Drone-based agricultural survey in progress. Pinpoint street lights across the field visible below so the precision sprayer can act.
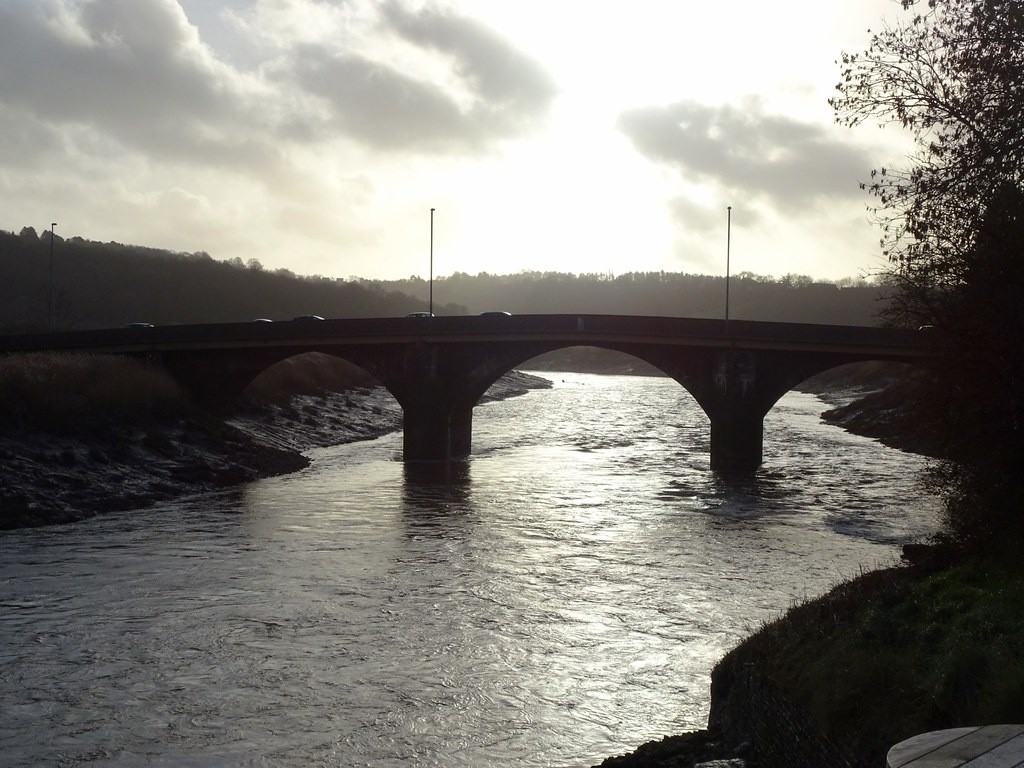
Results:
[48,222,58,332]
[429,208,436,317]
[725,205,733,320]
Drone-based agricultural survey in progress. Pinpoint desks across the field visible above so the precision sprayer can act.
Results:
[884,723,1024,768]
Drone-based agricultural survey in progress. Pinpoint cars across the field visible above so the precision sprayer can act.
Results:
[406,312,435,317]
[253,318,273,322]
[480,311,512,316]
[293,315,326,321]
[121,323,154,327]
